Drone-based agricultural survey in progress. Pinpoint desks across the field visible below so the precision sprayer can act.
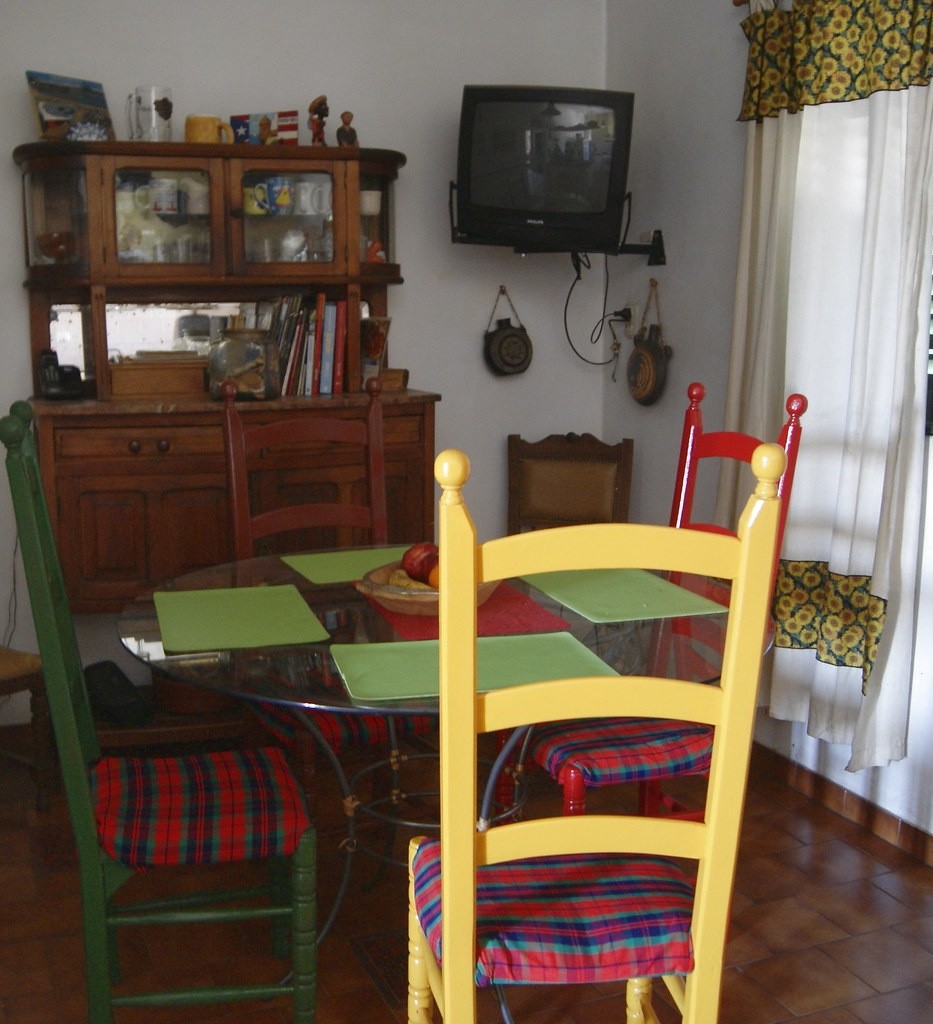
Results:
[113,544,779,1024]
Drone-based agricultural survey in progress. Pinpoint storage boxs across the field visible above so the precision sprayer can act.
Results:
[109,356,208,396]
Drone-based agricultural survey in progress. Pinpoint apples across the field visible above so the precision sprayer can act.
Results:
[401,541,438,585]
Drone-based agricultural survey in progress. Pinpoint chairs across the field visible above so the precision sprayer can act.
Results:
[495,381,809,826]
[219,377,438,821]
[405,441,789,1024]
[508,432,635,537]
[0,400,318,1024]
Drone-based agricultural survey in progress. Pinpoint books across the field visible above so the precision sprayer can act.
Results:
[261,293,393,401]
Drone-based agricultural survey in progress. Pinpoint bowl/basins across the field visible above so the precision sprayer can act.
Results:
[356,559,503,616]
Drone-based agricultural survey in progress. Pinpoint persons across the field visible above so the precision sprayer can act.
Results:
[336,111,359,147]
[308,94,329,146]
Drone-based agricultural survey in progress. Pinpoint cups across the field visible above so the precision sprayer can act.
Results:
[255,238,273,262]
[185,115,234,145]
[360,190,382,215]
[254,176,294,215]
[243,186,268,214]
[126,84,173,142]
[141,231,155,262]
[170,237,190,262]
[199,231,211,263]
[312,182,333,213]
[135,179,178,214]
[291,182,318,215]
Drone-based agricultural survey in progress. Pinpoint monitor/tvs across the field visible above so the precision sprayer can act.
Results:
[454,84,635,249]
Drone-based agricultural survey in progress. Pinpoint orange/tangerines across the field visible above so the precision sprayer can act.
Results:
[387,570,434,590]
[428,565,439,590]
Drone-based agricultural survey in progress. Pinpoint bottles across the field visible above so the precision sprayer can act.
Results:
[323,211,333,260]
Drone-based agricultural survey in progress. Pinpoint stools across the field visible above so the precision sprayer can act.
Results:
[0,645,52,812]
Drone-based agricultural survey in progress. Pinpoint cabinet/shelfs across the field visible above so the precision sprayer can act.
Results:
[11,139,443,794]
